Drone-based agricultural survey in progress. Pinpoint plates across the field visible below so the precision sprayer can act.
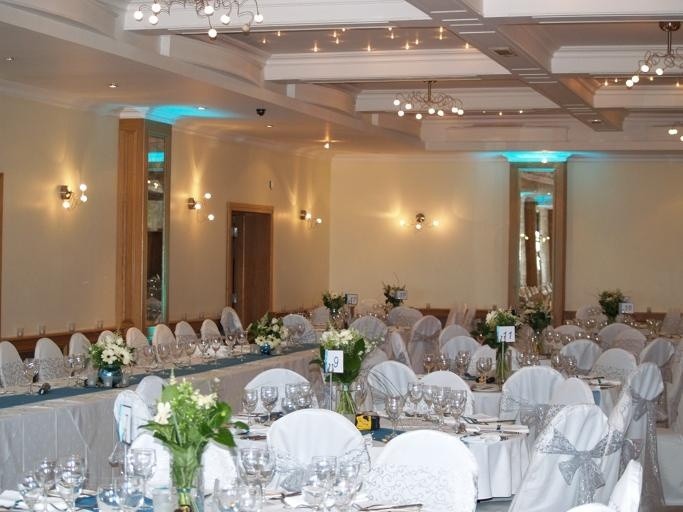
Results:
[457,432,500,444]
[591,384,613,389]
[471,384,499,392]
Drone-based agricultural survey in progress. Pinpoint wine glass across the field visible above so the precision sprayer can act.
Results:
[517,332,542,368]
[127,331,249,380]
[422,350,493,384]
[15,440,176,511]
[0,353,86,397]
[239,381,314,427]
[625,315,662,339]
[211,445,361,511]
[384,381,467,441]
[284,320,304,352]
[546,329,601,359]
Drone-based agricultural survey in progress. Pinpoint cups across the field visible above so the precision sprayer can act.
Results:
[564,318,609,329]
[86,372,128,388]
[180,309,222,323]
[423,301,430,309]
[95,320,103,328]
[352,312,388,322]
[38,324,45,335]
[16,328,23,337]
[550,351,577,378]
[280,302,320,315]
[68,321,75,331]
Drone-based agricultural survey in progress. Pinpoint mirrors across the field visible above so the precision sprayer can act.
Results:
[516,166,554,322]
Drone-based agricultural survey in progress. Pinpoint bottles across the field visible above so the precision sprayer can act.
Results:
[330,382,361,425]
[331,307,348,330]
[259,342,270,354]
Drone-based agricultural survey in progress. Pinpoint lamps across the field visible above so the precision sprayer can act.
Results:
[393,80,463,121]
[398,213,439,232]
[134,0,262,39]
[59,181,87,211]
[619,21,682,88]
[187,191,214,224]
[300,209,321,232]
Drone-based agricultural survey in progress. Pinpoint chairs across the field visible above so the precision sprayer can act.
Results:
[0,288,682,512]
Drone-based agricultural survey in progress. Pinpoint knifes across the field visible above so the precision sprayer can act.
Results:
[363,504,423,511]
